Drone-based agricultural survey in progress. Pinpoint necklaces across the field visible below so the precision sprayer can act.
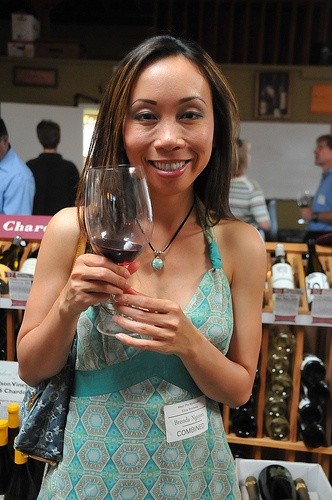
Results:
[135,199,198,273]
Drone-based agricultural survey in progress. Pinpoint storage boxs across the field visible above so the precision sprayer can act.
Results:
[7,40,44,59]
[11,13,41,41]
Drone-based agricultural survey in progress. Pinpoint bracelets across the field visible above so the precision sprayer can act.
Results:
[312,212,319,223]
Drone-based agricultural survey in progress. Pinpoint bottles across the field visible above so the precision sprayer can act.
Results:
[19,247,40,275]
[258,464,296,500]
[260,77,269,115]
[305,238,332,310]
[233,326,332,448]
[0,403,47,500]
[276,78,286,115]
[271,244,295,290]
[0,236,23,294]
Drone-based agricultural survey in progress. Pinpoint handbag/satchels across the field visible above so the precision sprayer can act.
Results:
[12,331,78,468]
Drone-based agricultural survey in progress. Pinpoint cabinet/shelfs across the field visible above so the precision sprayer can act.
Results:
[0,236,332,485]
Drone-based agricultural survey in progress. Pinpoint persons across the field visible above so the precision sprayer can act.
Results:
[297,135,332,273]
[229,136,272,243]
[0,116,36,217]
[25,119,80,215]
[14,31,272,500]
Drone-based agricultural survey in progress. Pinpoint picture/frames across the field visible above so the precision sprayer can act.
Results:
[254,70,293,119]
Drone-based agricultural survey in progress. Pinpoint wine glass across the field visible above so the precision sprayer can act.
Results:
[83,163,153,336]
[296,190,310,224]
